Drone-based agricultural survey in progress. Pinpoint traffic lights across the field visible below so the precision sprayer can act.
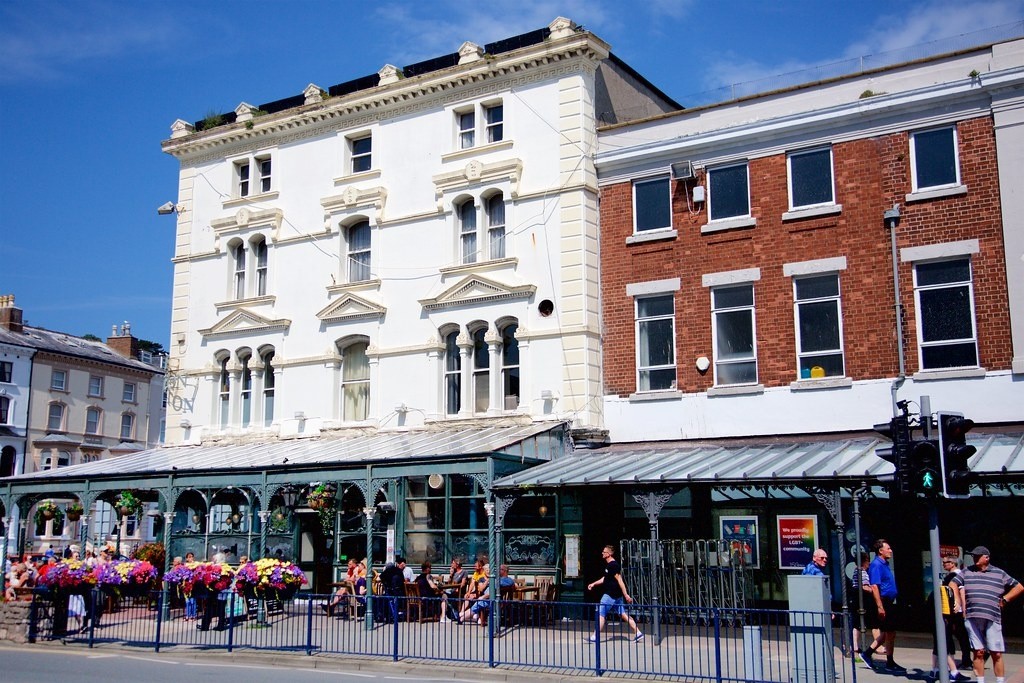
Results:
[938,411,978,499]
[873,415,910,498]
[912,439,944,496]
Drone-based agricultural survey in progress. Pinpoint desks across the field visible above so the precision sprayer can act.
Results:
[430,578,465,615]
[327,577,354,617]
[503,582,542,611]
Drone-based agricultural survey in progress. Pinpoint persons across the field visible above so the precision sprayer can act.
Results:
[582,544,644,643]
[848,538,906,672]
[928,546,1024,683]
[801,549,840,679]
[320,557,515,626]
[4,544,252,634]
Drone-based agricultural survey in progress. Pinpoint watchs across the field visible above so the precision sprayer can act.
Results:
[1001,594,1009,605]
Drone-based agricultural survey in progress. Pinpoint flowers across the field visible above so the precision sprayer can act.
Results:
[310,488,335,516]
[115,490,141,507]
[92,552,158,603]
[32,498,64,522]
[28,548,91,612]
[170,557,234,605]
[237,557,303,603]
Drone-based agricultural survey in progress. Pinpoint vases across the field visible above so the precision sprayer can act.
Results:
[116,503,137,518]
[43,511,55,520]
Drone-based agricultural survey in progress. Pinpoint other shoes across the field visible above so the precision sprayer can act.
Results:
[441,616,451,622]
[213,626,225,631]
[957,662,973,671]
[834,671,841,677]
[452,608,463,625]
[196,624,209,631]
[853,648,862,654]
[185,617,198,622]
[320,603,335,616]
[875,646,886,654]
[79,626,88,634]
[478,618,481,624]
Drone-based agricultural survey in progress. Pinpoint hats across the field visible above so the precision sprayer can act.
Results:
[968,546,990,555]
[99,545,109,552]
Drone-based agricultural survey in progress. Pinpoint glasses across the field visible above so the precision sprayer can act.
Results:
[943,561,951,564]
[239,561,244,563]
[971,554,983,557]
[603,550,608,554]
[76,555,81,558]
[816,556,828,560]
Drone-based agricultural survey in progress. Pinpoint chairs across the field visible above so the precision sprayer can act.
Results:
[530,573,556,609]
[505,573,529,586]
[403,578,427,627]
[427,573,445,583]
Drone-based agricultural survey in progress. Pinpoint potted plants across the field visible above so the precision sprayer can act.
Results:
[62,503,86,519]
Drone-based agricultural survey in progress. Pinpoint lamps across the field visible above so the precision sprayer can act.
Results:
[670,159,707,216]
[281,482,304,517]
[158,200,184,218]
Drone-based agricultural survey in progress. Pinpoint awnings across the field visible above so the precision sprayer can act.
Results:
[492,426,1024,500]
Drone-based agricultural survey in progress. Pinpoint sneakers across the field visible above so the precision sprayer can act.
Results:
[949,672,971,682]
[584,636,597,643]
[929,670,951,678]
[885,661,907,673]
[633,633,644,643]
[858,652,874,669]
